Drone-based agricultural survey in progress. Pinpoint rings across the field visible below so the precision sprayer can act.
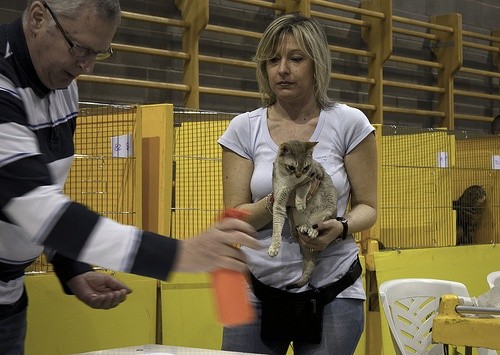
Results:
[306,194,312,200]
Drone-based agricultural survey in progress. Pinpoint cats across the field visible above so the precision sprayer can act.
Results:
[267,140,339,291]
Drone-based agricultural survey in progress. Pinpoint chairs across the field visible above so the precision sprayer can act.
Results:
[379,278,497,355]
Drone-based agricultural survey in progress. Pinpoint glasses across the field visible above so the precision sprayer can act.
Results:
[40,0,114,62]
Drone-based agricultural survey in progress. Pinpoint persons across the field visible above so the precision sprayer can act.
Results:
[0,0,266,355]
[217,14,378,355]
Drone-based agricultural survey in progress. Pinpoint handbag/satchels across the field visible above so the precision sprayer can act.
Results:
[241,255,362,346]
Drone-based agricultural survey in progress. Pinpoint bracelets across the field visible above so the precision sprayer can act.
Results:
[336,217,348,239]
[265,191,275,214]
